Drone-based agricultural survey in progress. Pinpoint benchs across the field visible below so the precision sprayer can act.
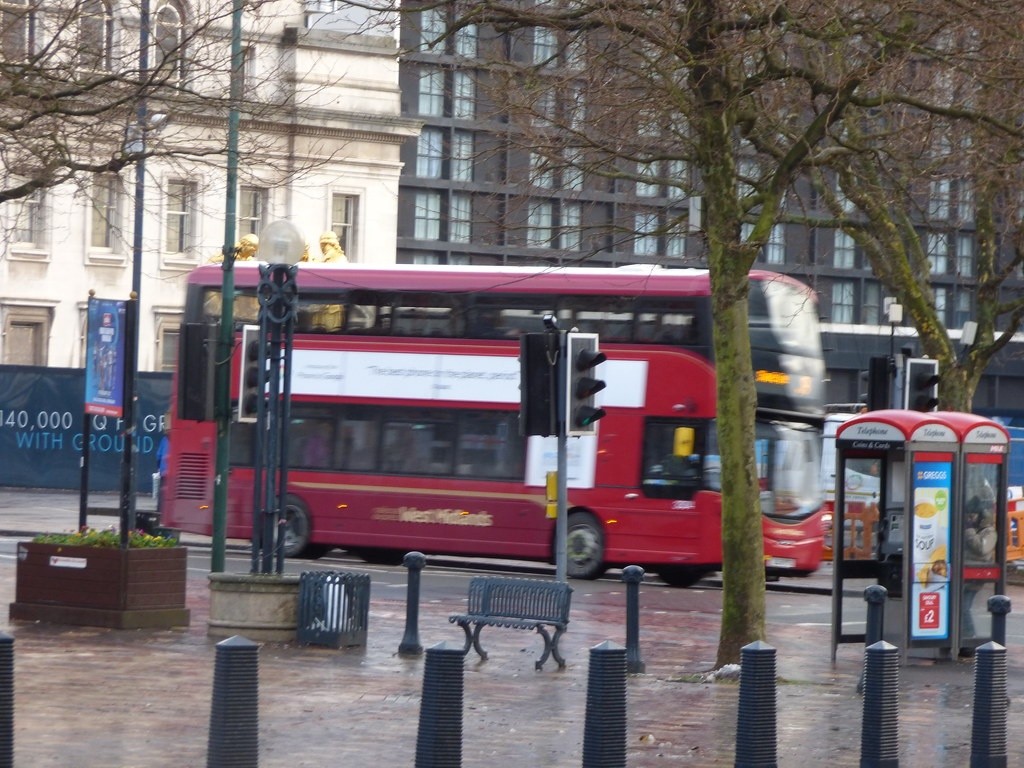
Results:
[450,576,573,670]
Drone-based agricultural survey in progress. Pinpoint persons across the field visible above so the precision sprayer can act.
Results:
[661,452,700,485]
[154,435,170,509]
[970,471,994,510]
[209,230,348,263]
[939,495,998,657]
[301,424,334,467]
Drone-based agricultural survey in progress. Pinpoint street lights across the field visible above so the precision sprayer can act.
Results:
[883,297,904,408]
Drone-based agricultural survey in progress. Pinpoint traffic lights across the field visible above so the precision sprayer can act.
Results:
[862,357,887,412]
[904,360,942,414]
[564,330,609,437]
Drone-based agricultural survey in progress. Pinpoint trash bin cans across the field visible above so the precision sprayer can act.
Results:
[298,570,371,648]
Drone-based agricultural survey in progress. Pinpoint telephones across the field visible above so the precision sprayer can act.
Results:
[877,510,904,554]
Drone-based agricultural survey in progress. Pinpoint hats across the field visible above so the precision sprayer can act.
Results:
[964,495,982,513]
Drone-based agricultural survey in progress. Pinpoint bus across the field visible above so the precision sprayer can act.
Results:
[155,265,829,588]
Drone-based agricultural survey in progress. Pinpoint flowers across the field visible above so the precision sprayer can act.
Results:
[32,525,179,548]
